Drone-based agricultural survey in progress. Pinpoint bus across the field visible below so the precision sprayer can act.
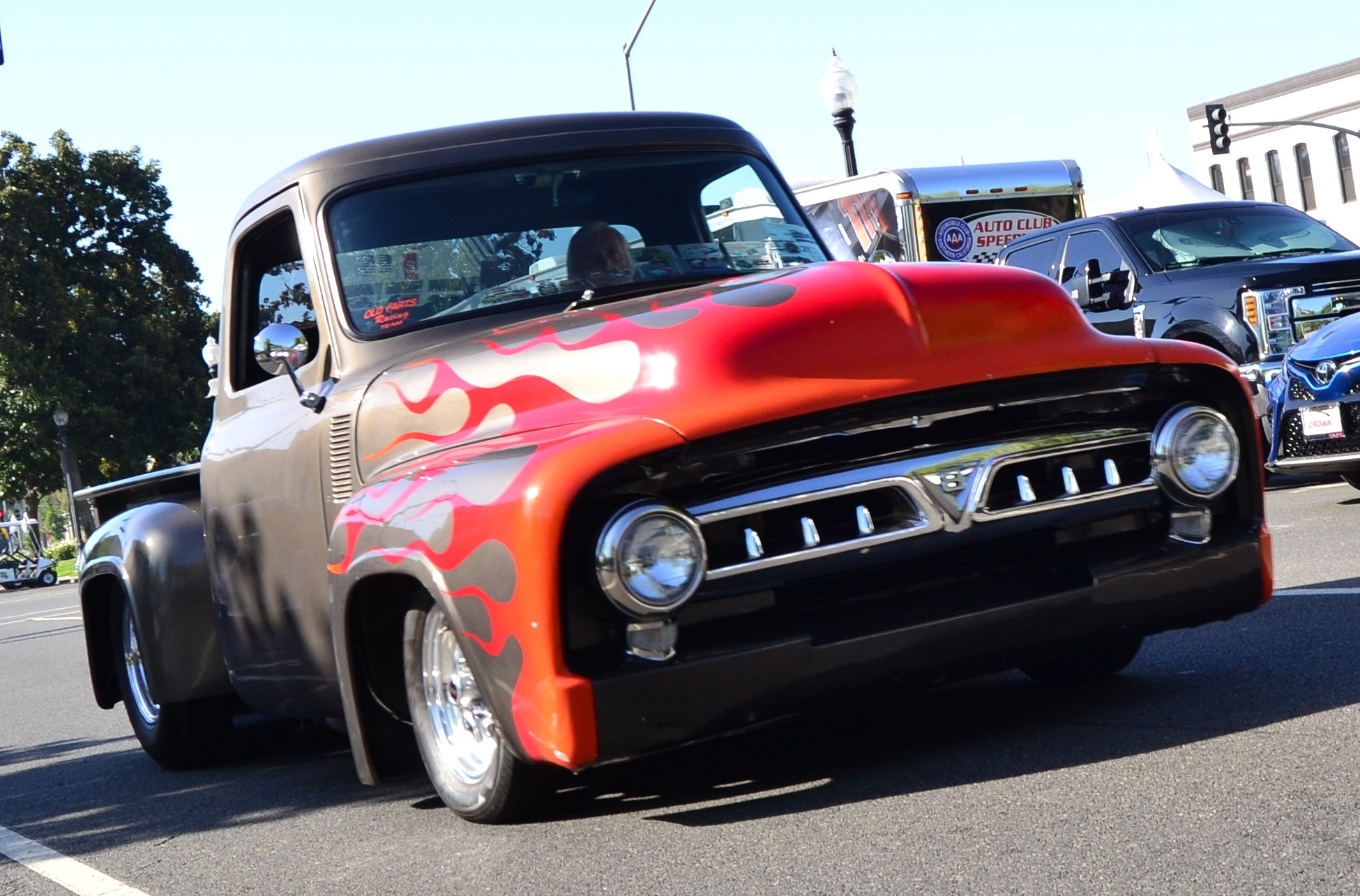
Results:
[699,157,1087,278]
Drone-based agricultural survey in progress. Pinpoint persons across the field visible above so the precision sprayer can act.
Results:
[568,223,636,294]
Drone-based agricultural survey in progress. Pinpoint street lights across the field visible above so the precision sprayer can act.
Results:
[823,48,861,179]
[51,397,85,553]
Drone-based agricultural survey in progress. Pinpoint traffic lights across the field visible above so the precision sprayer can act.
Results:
[1203,104,1231,156]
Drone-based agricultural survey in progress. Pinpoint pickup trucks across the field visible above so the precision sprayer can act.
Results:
[77,109,1275,823]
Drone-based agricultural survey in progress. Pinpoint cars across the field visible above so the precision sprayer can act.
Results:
[995,200,1360,366]
[1264,313,1360,490]
